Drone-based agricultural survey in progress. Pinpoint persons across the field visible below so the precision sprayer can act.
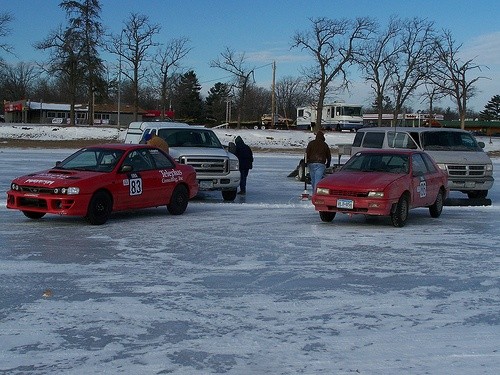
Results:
[143,132,169,155]
[303,131,332,194]
[228,136,253,194]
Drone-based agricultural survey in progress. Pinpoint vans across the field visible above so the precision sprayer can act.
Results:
[350,126,494,199]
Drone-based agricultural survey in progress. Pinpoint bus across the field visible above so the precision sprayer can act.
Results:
[311,103,364,132]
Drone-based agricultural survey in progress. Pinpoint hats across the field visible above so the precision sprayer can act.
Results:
[316,131,325,140]
[144,133,152,142]
[235,135,244,144]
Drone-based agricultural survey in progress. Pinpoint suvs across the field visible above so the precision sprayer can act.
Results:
[126,121,241,201]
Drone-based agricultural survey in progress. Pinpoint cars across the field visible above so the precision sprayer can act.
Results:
[6,144,201,225]
[261,114,293,124]
[312,148,449,228]
